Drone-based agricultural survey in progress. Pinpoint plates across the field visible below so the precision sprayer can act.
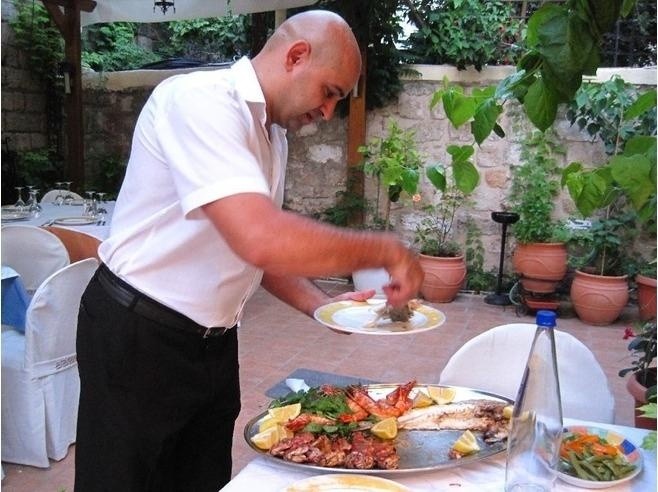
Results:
[243,383,534,474]
[280,473,414,492]
[312,296,446,334]
[531,424,645,488]
[1,208,30,221]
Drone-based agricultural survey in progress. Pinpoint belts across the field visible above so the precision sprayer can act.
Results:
[96,268,236,338]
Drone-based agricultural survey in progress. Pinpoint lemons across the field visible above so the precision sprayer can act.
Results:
[427,386,455,404]
[268,402,301,423]
[412,391,432,407]
[370,417,398,440]
[250,419,294,449]
[453,429,479,454]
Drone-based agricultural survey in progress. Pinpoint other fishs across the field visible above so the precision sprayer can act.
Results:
[397,399,536,444]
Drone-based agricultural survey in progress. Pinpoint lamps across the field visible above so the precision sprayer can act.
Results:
[153,0,176,16]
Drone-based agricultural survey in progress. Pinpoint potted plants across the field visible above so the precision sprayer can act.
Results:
[308,117,427,299]
[634,241,657,326]
[553,79,657,327]
[409,165,467,303]
[501,126,592,295]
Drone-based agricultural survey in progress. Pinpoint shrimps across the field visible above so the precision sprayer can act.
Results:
[284,414,336,432]
[320,379,417,422]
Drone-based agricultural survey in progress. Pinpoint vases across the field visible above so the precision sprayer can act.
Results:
[627,368,657,432]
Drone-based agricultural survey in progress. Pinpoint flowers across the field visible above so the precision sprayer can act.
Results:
[616,317,657,385]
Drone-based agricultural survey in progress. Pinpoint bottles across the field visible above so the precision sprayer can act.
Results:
[505,309,561,490]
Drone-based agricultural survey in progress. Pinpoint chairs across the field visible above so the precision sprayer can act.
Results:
[1,256,99,467]
[40,189,84,203]
[37,225,105,264]
[1,225,71,306]
[439,323,615,424]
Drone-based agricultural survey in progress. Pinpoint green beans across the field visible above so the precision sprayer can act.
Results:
[559,433,637,481]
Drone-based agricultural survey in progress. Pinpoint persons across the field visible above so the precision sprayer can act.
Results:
[75,9,425,490]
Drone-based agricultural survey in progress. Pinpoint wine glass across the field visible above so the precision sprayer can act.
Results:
[13,179,108,225]
